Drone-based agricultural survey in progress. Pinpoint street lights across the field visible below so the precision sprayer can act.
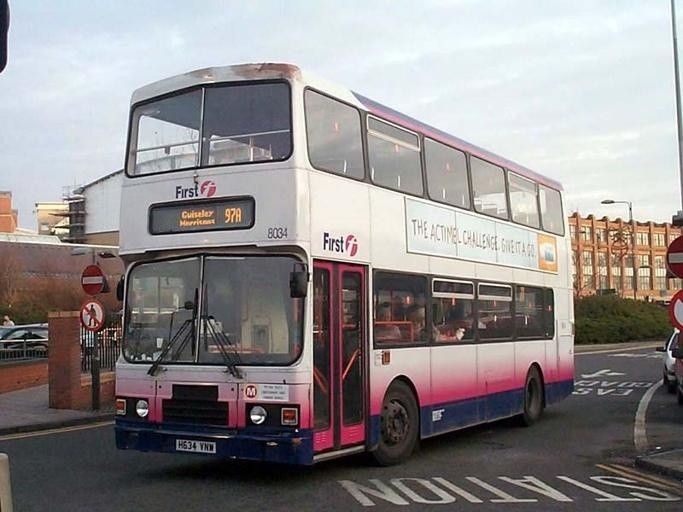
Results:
[602,198,637,299]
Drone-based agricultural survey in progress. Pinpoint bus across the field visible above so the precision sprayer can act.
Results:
[114,62,578,472]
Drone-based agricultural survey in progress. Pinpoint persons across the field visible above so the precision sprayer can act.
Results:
[373,302,400,340]
[2,315,14,326]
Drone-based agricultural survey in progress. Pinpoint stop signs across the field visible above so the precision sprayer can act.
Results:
[81,265,104,296]
[665,236,683,279]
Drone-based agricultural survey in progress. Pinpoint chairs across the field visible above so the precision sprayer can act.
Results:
[481,310,527,338]
[316,158,552,232]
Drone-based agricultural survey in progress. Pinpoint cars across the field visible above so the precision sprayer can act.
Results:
[1,323,98,358]
[656,330,682,401]
